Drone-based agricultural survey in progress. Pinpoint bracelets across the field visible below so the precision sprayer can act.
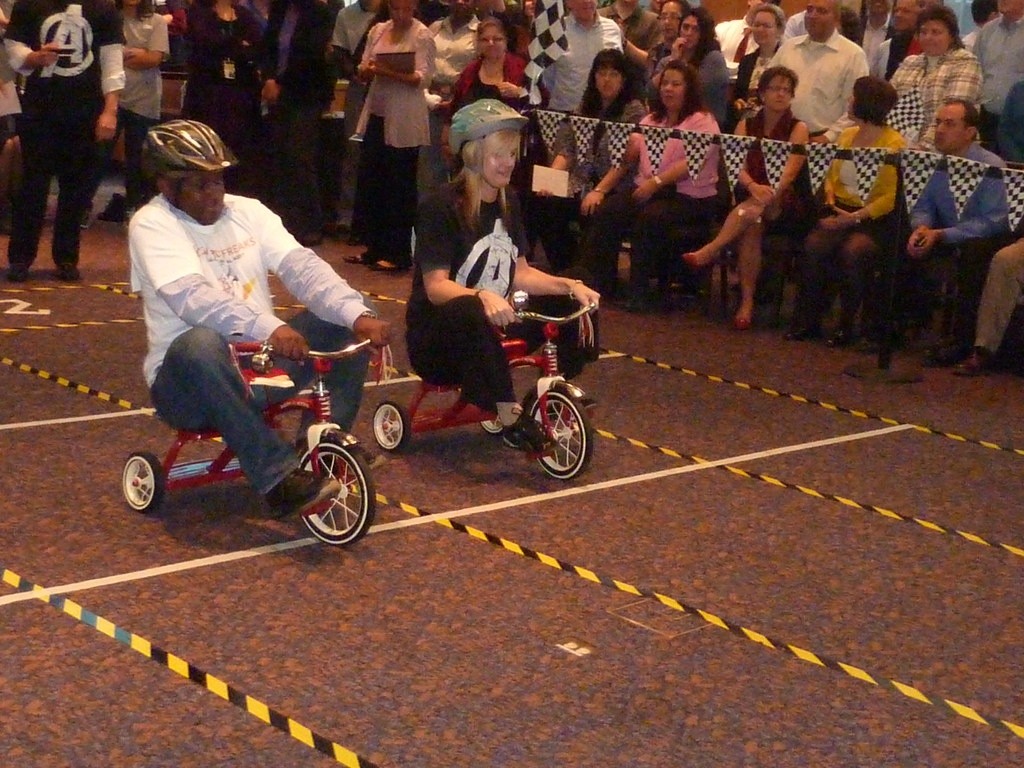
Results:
[592,188,608,195]
[654,176,664,188]
[475,289,483,296]
[746,180,754,188]
[854,211,861,224]
[569,279,583,299]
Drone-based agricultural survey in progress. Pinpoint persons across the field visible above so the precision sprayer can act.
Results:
[0,0,1024,381]
[404,97,602,454]
[127,117,393,517]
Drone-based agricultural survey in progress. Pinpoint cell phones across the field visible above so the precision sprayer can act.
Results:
[50,48,75,54]
[916,236,927,247]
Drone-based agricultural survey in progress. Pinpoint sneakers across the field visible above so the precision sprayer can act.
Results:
[267,471,341,521]
[319,445,376,473]
[501,407,551,451]
[583,398,598,410]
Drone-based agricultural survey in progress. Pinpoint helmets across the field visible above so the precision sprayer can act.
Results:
[449,98,529,154]
[142,119,238,176]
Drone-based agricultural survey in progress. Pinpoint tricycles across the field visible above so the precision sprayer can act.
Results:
[122,338,377,547]
[372,290,597,480]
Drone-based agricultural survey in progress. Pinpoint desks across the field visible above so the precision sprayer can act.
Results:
[113,71,454,230]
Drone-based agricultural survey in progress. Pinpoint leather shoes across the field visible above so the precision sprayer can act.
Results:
[784,325,815,341]
[826,325,852,347]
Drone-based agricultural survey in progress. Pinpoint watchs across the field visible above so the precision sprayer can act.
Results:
[361,312,376,319]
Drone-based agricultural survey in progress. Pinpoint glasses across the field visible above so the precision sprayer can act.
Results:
[753,20,778,30]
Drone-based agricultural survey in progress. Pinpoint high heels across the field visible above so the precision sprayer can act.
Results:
[682,250,721,273]
[736,299,756,330]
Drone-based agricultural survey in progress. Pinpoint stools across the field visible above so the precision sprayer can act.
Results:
[567,224,1024,373]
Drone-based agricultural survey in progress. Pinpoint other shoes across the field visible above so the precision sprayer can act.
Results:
[7,262,28,281]
[302,230,323,246]
[349,234,365,248]
[58,262,80,281]
[371,260,408,270]
[342,252,371,264]
[80,209,92,228]
[952,347,997,375]
[0,205,12,234]
[923,345,951,365]
